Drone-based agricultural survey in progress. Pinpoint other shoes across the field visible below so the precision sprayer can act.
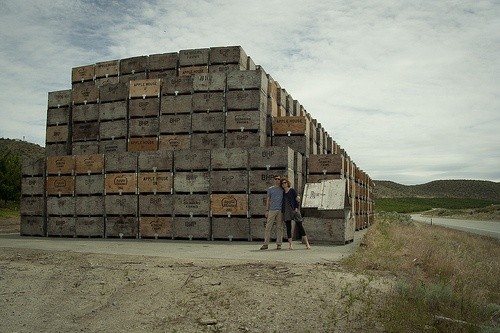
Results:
[306,244,311,249]
[277,244,281,249]
[290,245,294,249]
[260,245,268,250]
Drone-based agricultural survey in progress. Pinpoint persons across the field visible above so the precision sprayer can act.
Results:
[259,174,300,249]
[279,177,312,250]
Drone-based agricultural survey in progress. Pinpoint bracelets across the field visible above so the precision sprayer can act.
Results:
[267,210,269,211]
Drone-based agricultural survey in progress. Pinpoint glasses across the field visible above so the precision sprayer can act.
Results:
[281,182,287,185]
[274,178,280,180]
